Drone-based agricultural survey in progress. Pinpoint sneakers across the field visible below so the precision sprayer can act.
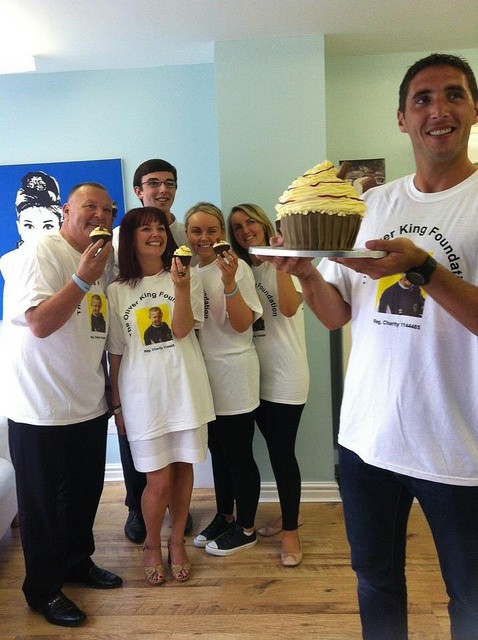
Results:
[193,513,235,548]
[205,523,257,556]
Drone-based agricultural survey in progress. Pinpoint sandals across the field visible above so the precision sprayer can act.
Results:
[167,539,191,583]
[142,540,165,585]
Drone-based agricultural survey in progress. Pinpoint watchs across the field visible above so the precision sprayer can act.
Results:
[406,254,437,286]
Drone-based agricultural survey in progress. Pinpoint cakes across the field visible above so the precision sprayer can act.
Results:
[212,237,230,258]
[111,200,117,217]
[89,224,112,248]
[172,244,192,266]
[275,160,366,250]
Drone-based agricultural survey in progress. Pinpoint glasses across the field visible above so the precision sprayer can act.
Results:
[138,179,176,188]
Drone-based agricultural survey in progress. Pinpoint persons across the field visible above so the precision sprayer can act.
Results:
[91,294,105,332]
[226,203,311,565]
[15,170,64,248]
[106,206,218,587]
[111,159,193,544]
[143,306,173,346]
[185,202,265,555]
[378,275,425,318]
[1,184,123,627]
[256,53,478,640]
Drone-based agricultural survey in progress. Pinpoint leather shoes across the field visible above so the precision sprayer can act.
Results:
[28,593,86,627]
[68,557,122,589]
[256,515,303,537]
[280,530,302,567]
[183,512,193,535]
[124,507,146,543]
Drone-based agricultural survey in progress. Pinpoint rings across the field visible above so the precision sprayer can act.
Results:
[179,271,184,277]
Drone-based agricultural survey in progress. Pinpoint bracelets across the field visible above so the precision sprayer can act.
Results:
[223,285,239,298]
[111,405,122,416]
[71,273,91,294]
